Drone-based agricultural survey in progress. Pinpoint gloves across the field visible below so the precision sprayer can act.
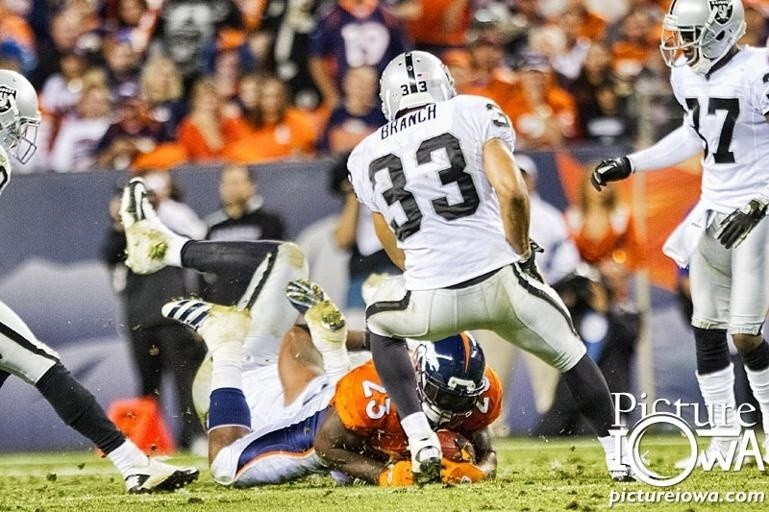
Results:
[713,197,765,250]
[590,158,629,192]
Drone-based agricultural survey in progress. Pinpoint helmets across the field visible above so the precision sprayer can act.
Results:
[378,50,456,122]
[658,0,746,77]
[0,69,42,166]
[411,330,490,434]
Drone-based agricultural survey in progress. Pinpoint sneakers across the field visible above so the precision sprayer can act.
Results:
[161,294,253,352]
[118,176,168,275]
[605,450,658,483]
[122,450,200,494]
[676,438,751,469]
[287,278,347,333]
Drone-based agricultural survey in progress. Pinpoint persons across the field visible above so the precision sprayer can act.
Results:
[2,0,769,496]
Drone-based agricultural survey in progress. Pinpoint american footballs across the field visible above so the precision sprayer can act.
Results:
[437,429,476,465]
